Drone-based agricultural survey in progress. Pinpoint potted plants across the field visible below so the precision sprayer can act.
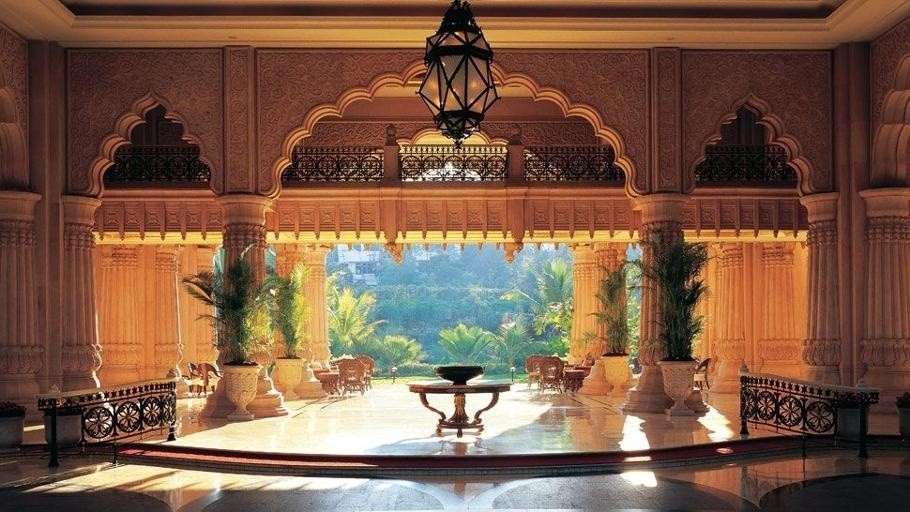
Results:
[0,401,25,453]
[895,392,909,439]
[581,256,642,397]
[46,410,82,447]
[269,262,306,401]
[637,225,710,416]
[835,395,869,444]
[183,260,276,421]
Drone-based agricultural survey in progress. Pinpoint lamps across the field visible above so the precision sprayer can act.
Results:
[414,0,502,150]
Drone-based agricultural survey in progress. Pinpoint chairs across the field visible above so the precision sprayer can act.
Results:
[187,362,212,393]
[196,363,222,395]
[526,354,595,397]
[315,356,374,397]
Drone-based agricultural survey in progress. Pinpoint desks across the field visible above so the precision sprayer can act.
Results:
[408,380,514,438]
